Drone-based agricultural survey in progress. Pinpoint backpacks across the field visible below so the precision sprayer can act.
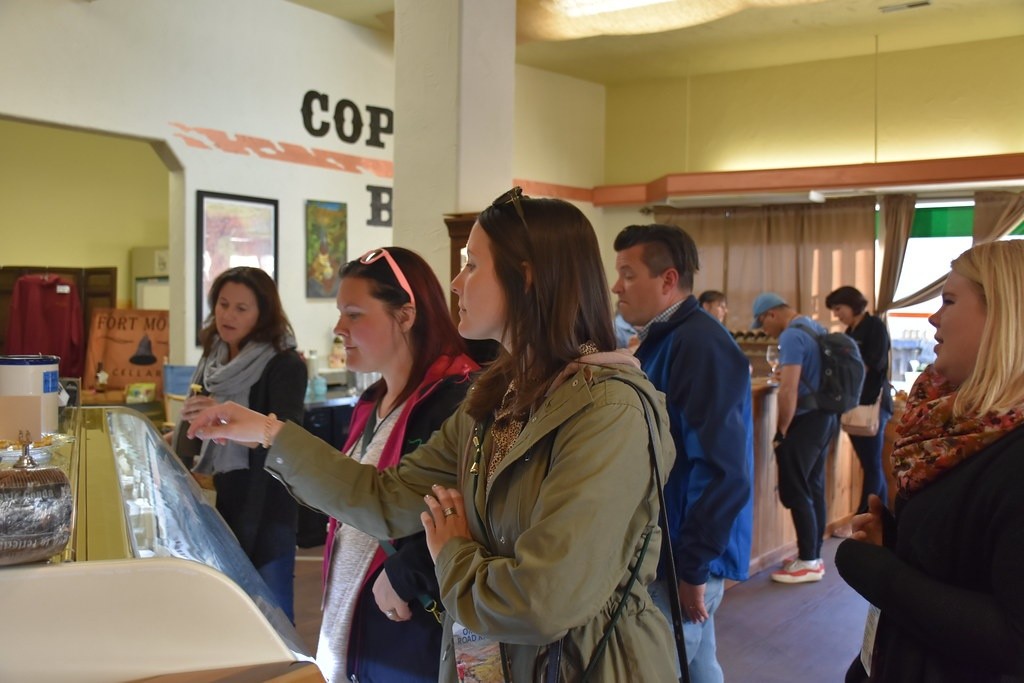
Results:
[778,323,865,414]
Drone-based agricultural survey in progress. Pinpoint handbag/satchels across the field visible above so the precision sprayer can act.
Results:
[840,400,880,436]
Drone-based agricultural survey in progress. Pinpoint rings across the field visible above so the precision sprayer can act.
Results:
[384,609,396,619]
[689,604,697,610]
[440,504,457,519]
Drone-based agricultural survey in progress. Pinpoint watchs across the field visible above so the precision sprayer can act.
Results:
[772,428,786,444]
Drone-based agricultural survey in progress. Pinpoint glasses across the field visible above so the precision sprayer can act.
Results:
[360,247,416,305]
[492,185,529,229]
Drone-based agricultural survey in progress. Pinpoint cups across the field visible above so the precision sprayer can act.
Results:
[1,354,59,434]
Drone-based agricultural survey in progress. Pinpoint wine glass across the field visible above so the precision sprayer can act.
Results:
[766,344,783,386]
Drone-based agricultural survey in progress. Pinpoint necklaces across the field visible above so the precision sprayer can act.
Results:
[350,397,395,460]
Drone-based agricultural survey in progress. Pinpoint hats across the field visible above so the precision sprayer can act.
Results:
[750,292,787,328]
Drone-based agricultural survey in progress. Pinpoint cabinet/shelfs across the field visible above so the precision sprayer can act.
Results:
[296,406,352,550]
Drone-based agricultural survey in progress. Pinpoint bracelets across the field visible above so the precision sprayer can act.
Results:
[262,410,280,450]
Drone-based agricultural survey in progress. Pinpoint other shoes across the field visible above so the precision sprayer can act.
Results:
[771,557,825,583]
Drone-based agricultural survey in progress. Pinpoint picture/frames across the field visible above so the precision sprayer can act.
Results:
[196,189,279,347]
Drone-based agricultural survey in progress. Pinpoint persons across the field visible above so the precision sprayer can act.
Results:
[699,290,729,326]
[166,267,307,630]
[826,240,1024,683]
[183,186,682,683]
[309,247,486,683]
[750,292,840,583]
[612,223,756,683]
[614,314,643,349]
[825,286,897,537]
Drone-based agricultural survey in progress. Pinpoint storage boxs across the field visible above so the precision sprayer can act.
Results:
[126,383,155,404]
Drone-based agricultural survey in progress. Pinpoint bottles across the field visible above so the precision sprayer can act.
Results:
[299,349,327,401]
